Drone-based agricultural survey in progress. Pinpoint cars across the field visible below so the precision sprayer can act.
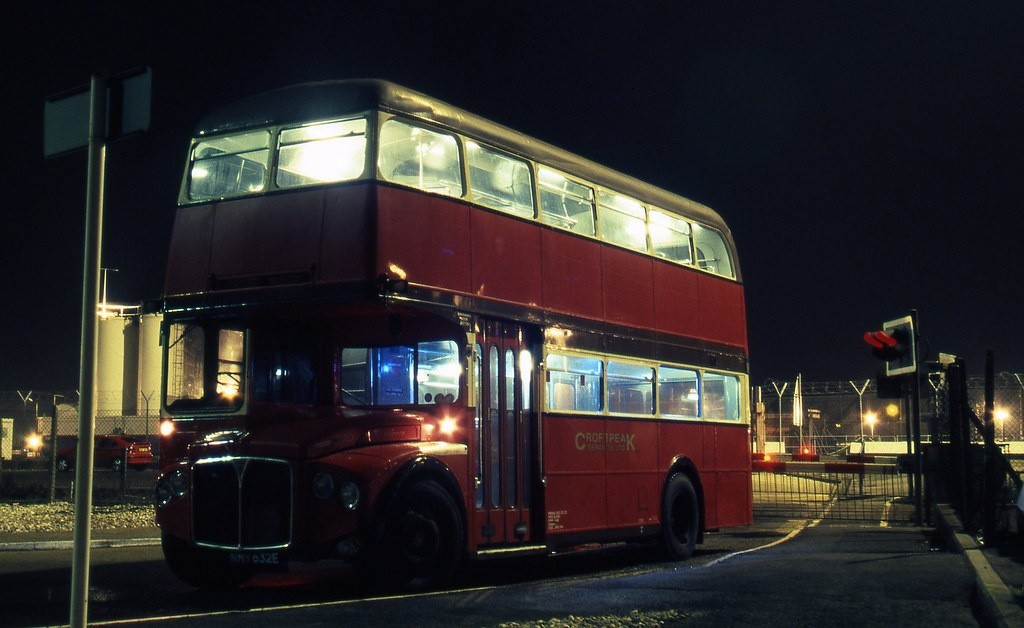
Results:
[48,435,154,474]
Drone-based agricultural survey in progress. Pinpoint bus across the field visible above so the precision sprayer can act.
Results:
[152,75,753,596]
